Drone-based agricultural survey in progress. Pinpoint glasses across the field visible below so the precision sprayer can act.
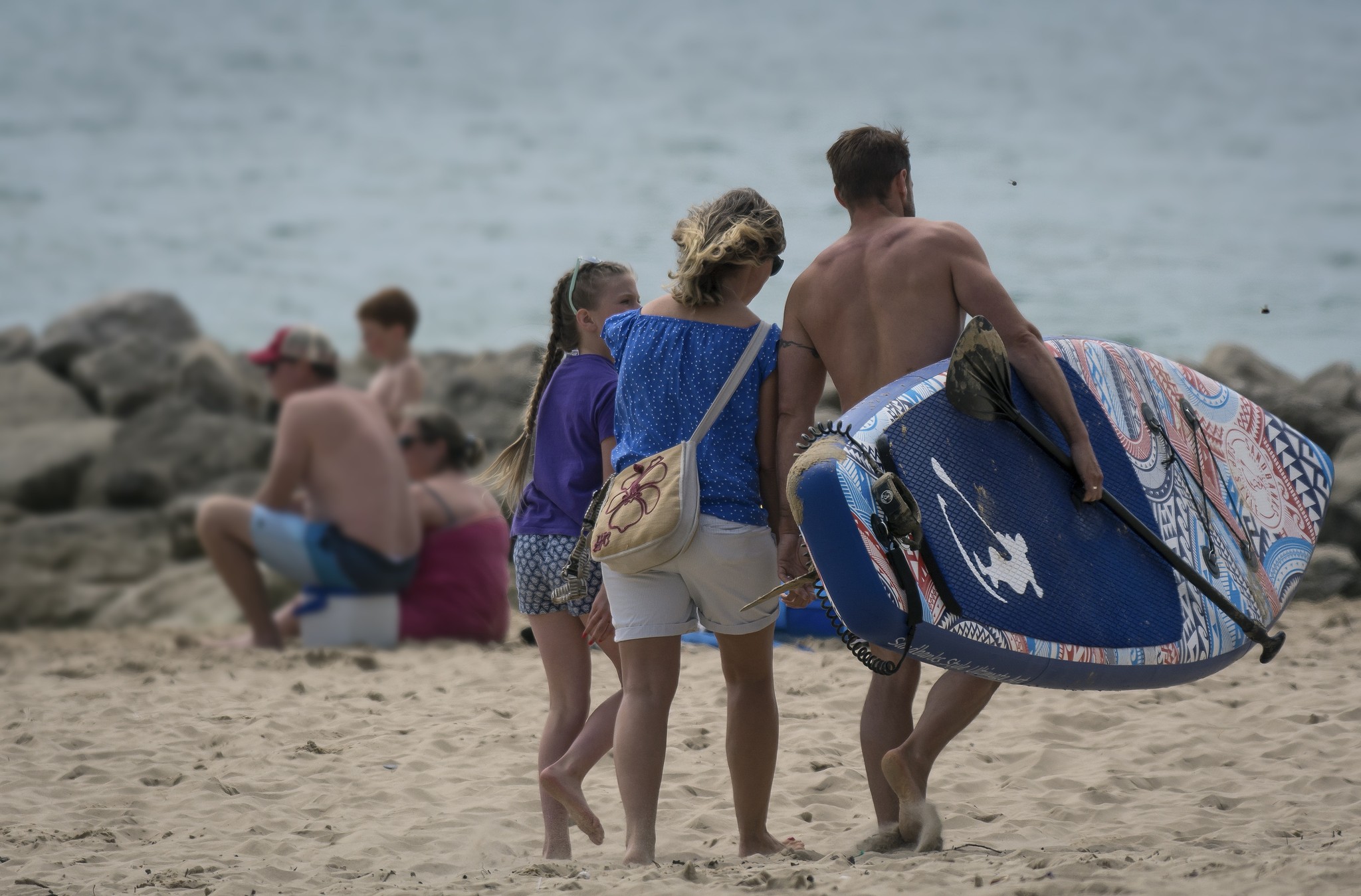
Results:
[266,362,277,376]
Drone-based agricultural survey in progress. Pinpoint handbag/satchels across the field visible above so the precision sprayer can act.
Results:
[588,441,698,575]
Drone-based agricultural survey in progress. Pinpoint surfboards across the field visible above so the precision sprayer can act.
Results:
[784,338,1336,692]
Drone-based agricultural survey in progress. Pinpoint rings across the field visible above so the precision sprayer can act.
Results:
[1092,485,1099,490]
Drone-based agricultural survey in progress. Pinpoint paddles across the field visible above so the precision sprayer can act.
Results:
[944,315,1287,664]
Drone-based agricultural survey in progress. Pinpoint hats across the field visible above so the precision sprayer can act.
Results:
[247,326,338,367]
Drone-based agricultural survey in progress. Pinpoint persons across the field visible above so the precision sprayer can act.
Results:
[509,261,641,860]
[601,188,803,861]
[777,127,1103,845]
[197,326,422,653]
[356,288,430,433]
[399,411,509,646]
